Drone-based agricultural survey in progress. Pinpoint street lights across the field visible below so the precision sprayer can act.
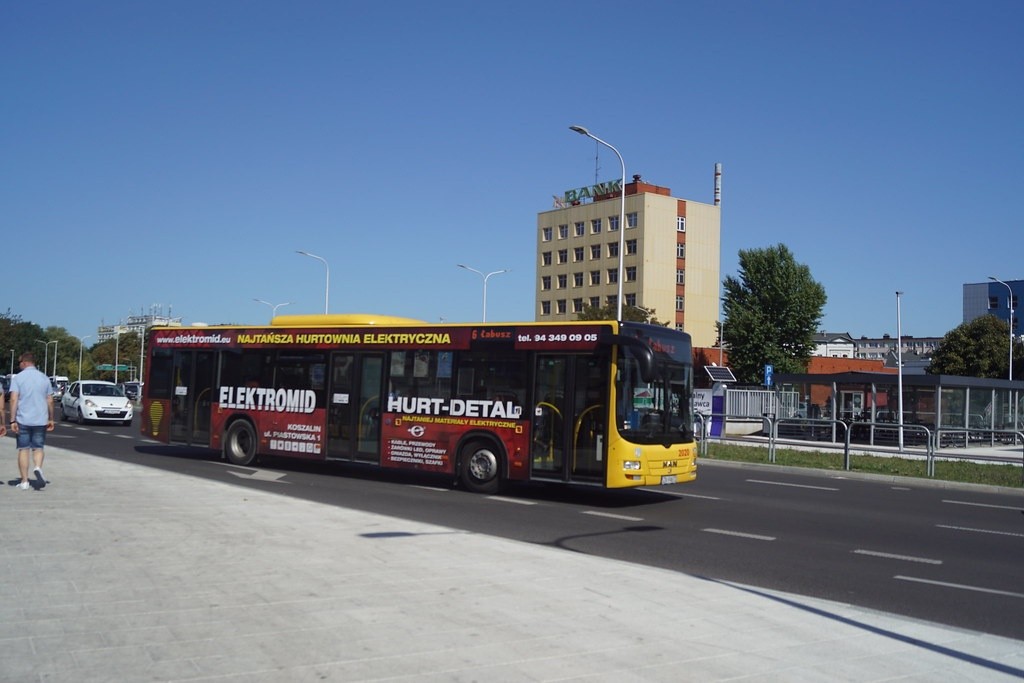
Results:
[457,262,513,326]
[34,339,59,377]
[894,290,904,453]
[69,334,92,381]
[986,276,1014,381]
[9,349,16,376]
[252,298,291,318]
[568,124,627,321]
[295,249,330,315]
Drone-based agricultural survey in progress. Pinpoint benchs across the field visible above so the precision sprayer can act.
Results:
[776,419,1024,449]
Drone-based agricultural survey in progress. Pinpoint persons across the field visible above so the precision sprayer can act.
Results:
[0,352,55,490]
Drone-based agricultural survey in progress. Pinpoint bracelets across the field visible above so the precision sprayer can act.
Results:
[9,421,16,424]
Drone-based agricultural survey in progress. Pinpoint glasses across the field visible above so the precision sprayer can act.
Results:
[17,360,22,364]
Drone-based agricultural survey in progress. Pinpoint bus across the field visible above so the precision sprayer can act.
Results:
[136,314,698,496]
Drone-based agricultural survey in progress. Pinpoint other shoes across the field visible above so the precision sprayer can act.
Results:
[33,467,47,488]
[15,482,30,490]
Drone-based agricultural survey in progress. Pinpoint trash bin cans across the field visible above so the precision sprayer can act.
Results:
[763,412,775,437]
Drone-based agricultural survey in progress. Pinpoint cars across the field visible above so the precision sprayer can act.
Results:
[125,381,143,401]
[60,379,135,426]
[0,374,70,402]
